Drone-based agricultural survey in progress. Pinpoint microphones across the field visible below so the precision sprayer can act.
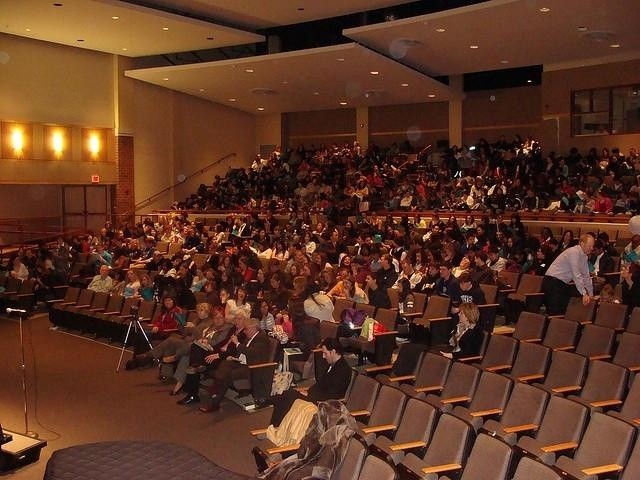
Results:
[6,307,26,314]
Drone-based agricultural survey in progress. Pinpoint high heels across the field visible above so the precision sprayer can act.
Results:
[168,383,185,396]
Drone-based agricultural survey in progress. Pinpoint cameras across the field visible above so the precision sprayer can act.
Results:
[129,305,139,315]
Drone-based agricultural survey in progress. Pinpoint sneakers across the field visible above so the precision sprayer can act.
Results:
[158,373,171,382]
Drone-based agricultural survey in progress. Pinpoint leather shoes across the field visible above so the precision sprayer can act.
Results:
[199,404,221,413]
[155,355,178,366]
[176,392,201,407]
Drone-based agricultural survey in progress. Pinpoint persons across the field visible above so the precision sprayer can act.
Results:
[271,338,352,427]
[2,133,639,410]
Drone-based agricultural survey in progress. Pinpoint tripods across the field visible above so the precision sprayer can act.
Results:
[116,315,161,372]
